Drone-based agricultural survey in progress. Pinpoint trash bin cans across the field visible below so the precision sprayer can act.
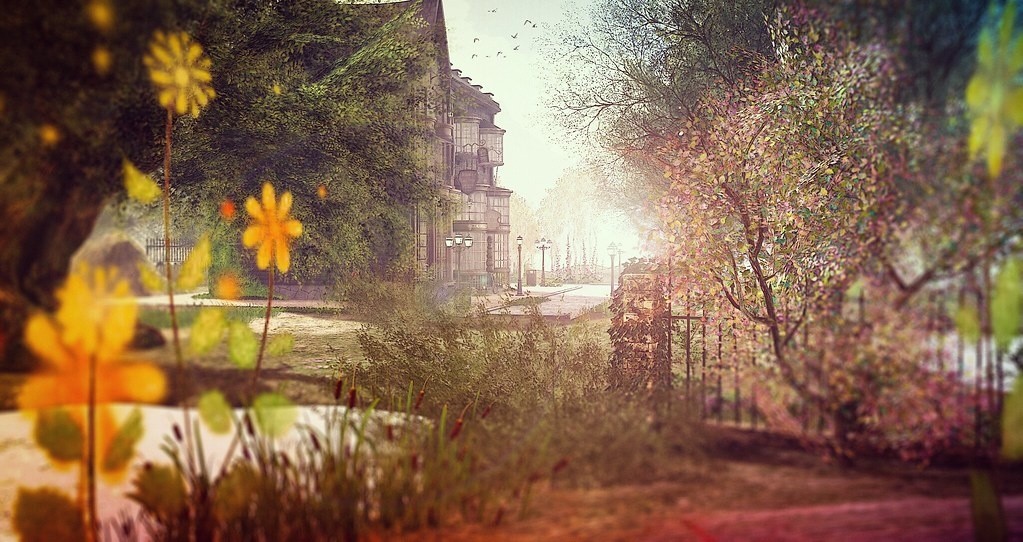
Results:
[526,269,536,286]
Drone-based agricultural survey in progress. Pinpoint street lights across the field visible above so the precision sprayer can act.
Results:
[515,235,524,296]
[445,233,473,291]
[534,238,552,286]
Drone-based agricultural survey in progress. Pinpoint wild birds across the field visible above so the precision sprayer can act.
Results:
[471,8,537,59]
